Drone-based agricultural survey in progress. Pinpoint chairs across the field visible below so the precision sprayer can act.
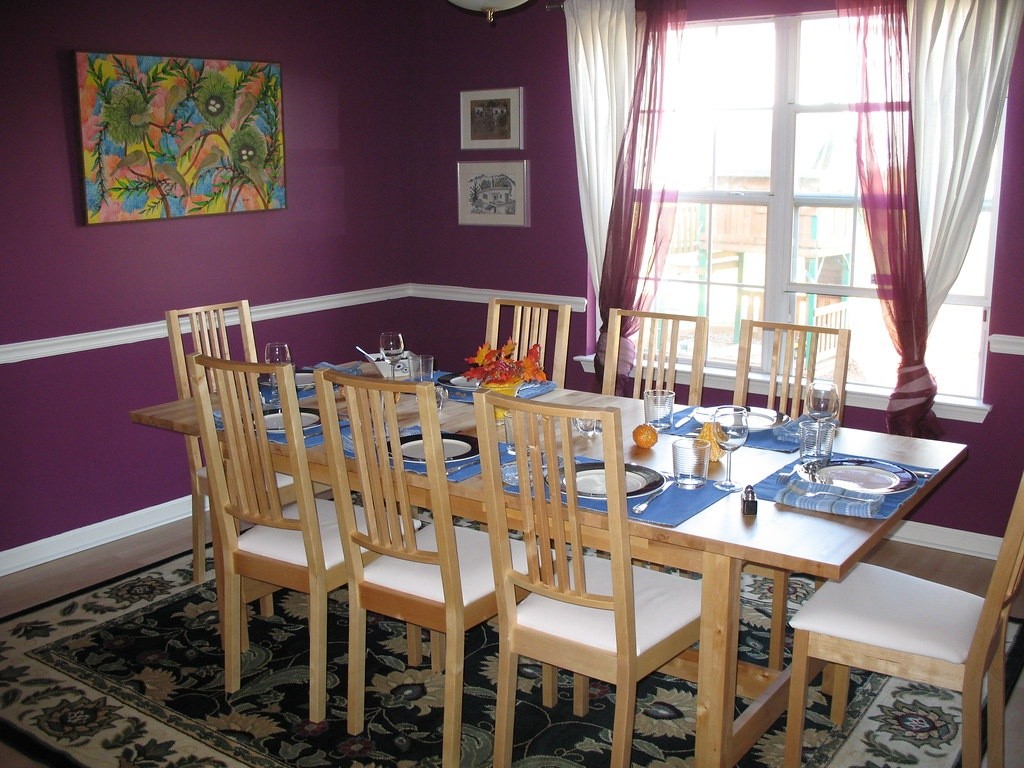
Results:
[732,319,851,668]
[602,308,711,570]
[165,300,334,619]
[314,367,558,768]
[478,297,573,533]
[474,386,703,768]
[188,352,422,723]
[783,471,1024,768]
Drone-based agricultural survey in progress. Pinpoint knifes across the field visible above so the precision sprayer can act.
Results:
[631,480,674,514]
[302,424,504,476]
[675,407,703,428]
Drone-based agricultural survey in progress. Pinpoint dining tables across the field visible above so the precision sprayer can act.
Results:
[128,359,968,768]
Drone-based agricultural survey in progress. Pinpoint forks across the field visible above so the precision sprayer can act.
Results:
[776,464,801,483]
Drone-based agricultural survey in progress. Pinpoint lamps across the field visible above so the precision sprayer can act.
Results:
[449,0,528,22]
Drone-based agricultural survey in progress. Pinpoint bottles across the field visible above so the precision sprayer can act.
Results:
[692,422,730,462]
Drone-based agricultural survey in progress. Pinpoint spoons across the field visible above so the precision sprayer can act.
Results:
[788,484,872,503]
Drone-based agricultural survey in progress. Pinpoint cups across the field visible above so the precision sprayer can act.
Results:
[503,411,530,456]
[673,438,710,490]
[644,389,675,430]
[408,355,434,381]
[572,417,602,438]
[416,387,448,412]
[799,421,837,474]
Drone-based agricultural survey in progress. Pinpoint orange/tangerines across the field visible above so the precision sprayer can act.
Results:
[633,424,658,448]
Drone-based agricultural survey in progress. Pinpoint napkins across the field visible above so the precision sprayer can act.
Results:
[303,362,363,377]
[772,415,842,443]
[516,381,554,400]
[213,401,259,427]
[501,451,579,487]
[774,479,887,519]
[341,424,417,454]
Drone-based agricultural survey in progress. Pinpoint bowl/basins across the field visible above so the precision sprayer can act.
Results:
[365,351,421,381]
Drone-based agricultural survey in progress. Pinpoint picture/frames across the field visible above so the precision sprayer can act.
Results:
[457,159,531,229]
[459,86,524,151]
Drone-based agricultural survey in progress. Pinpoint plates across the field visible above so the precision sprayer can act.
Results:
[257,370,339,389]
[438,373,478,391]
[797,458,918,494]
[387,433,480,463]
[693,406,792,432]
[544,461,666,500]
[252,407,322,433]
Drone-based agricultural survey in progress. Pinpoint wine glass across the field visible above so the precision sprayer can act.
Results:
[804,381,839,454]
[713,405,749,492]
[265,342,291,403]
[380,332,404,380]
